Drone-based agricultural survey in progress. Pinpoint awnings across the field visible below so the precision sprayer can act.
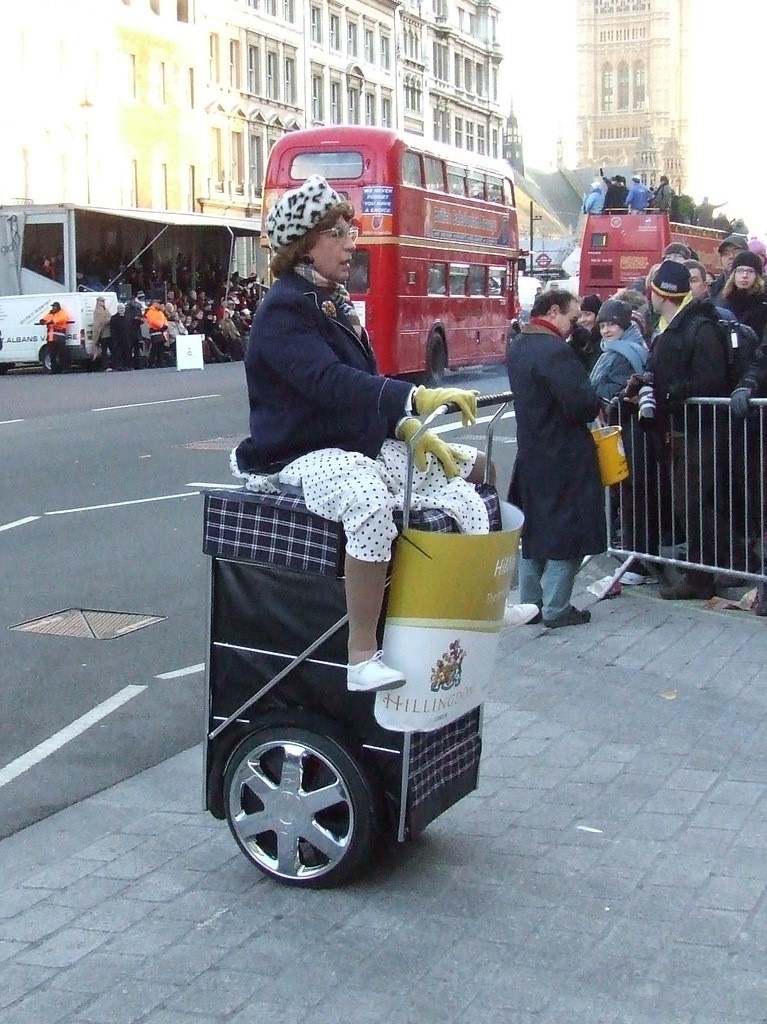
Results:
[78,206,261,291]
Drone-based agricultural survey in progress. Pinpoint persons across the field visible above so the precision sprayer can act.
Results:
[40,302,67,374]
[567,233,767,616]
[228,174,539,691]
[507,290,603,629]
[582,168,750,234]
[30,236,258,371]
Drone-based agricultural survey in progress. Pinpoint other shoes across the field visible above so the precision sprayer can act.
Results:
[660,583,712,602]
[105,367,113,372]
[718,559,761,586]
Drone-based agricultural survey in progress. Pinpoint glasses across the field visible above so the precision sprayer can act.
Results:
[734,268,756,274]
[319,226,359,243]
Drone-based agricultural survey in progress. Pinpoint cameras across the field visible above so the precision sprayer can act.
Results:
[636,382,656,424]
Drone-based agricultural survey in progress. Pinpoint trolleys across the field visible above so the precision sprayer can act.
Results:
[200,391,515,891]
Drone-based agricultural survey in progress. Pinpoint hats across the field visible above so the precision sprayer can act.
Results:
[590,183,599,190]
[596,299,632,330]
[663,241,692,261]
[265,174,342,252]
[747,237,766,255]
[732,251,762,276]
[51,302,60,307]
[651,261,691,296]
[718,235,749,253]
[96,296,105,300]
[580,295,602,313]
[632,174,641,183]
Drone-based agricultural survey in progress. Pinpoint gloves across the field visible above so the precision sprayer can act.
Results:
[416,384,480,428]
[731,390,750,418]
[399,419,470,479]
[600,168,603,177]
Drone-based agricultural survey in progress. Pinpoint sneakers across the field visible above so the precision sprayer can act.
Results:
[544,603,592,627]
[523,607,542,625]
[504,604,540,630]
[347,650,406,690]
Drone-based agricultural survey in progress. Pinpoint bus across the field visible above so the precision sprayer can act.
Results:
[258,126,531,385]
[579,206,747,303]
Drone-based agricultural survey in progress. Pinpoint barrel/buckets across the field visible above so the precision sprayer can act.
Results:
[591,424,629,486]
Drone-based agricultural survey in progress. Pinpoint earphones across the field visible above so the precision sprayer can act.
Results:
[302,254,314,265]
